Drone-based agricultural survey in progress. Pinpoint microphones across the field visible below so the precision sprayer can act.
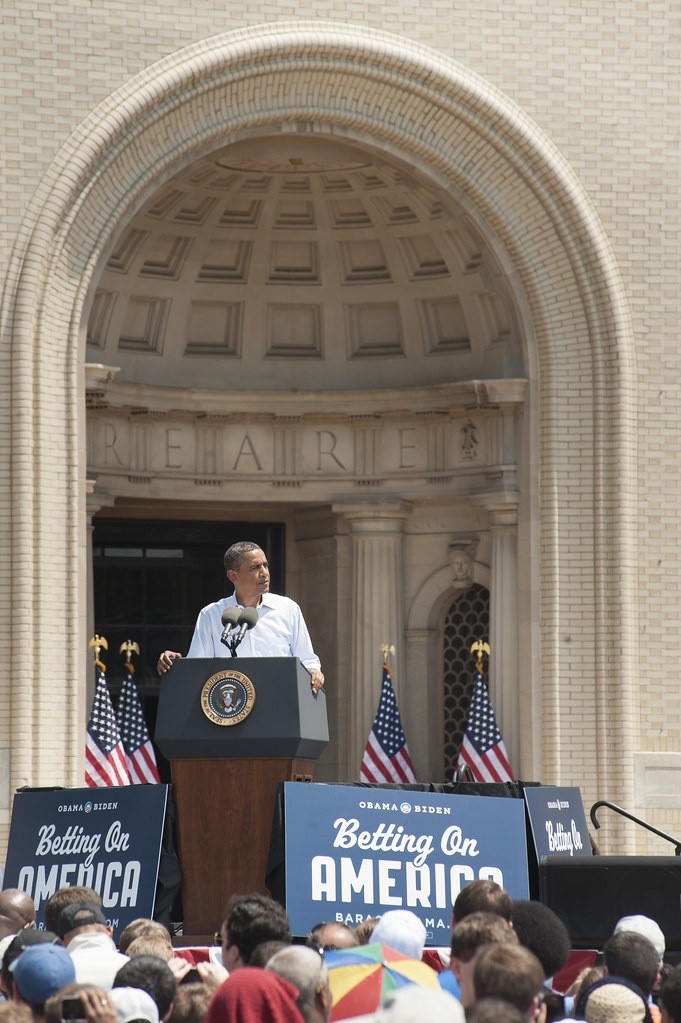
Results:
[221,606,241,641]
[237,607,259,641]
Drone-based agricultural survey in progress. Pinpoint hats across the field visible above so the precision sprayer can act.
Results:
[585,982,646,1023]
[7,941,76,1005]
[368,909,427,962]
[573,975,654,1023]
[108,986,160,1023]
[57,903,108,938]
[614,914,666,963]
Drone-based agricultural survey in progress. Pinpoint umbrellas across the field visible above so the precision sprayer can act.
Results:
[324,942,442,1023]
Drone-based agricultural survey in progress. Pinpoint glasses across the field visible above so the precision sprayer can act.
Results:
[311,921,352,936]
[214,933,236,947]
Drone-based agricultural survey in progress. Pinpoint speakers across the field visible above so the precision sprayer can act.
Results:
[539,855,681,950]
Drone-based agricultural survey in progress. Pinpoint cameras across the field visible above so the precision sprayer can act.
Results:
[61,996,91,1019]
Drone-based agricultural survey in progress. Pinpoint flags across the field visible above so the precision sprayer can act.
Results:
[83,661,160,788]
[359,664,418,783]
[453,663,514,783]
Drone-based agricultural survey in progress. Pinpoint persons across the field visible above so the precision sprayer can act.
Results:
[0,880,681,1023]
[157,542,324,694]
